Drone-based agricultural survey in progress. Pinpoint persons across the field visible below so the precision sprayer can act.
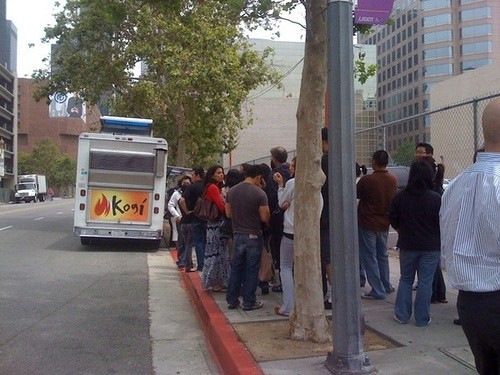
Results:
[453,148,485,325]
[438,98,500,375]
[164,143,448,327]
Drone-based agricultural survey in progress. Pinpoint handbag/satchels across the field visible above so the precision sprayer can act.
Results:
[258,242,275,282]
[193,182,221,223]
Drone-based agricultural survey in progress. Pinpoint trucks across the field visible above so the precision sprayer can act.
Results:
[15,174,46,203]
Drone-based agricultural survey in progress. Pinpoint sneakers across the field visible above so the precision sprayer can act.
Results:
[394,313,408,324]
[240,301,264,311]
[427,315,431,324]
[361,292,384,300]
[228,301,242,309]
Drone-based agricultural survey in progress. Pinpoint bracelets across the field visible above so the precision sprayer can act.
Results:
[278,186,284,187]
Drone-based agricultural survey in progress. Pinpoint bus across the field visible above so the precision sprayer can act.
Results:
[73,115,167,249]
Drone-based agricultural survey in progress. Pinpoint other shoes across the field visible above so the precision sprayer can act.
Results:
[186,268,196,272]
[192,263,196,267]
[274,306,290,317]
[179,265,186,269]
[213,287,228,293]
